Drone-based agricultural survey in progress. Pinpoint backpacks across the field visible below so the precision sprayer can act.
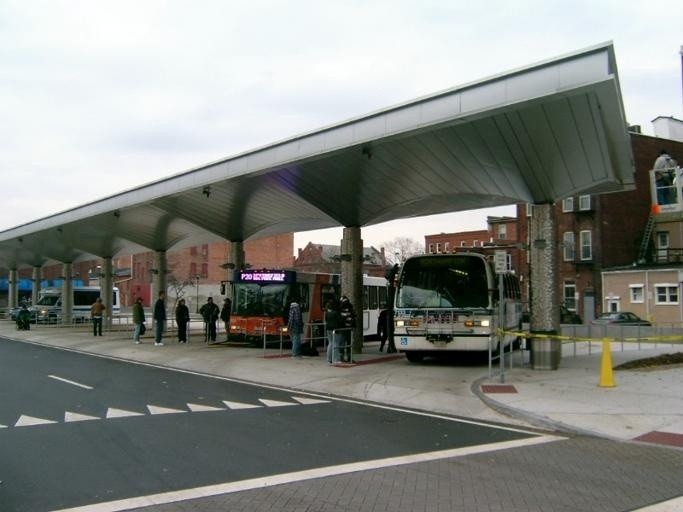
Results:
[139,325,146,335]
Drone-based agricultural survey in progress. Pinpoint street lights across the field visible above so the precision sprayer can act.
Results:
[196,274,199,313]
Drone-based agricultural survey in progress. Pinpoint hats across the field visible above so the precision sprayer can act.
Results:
[135,296,144,301]
[208,296,213,300]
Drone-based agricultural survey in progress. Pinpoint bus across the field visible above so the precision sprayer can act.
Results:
[221,268,389,345]
[393,252,523,361]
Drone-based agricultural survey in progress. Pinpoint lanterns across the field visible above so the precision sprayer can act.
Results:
[651,204,662,215]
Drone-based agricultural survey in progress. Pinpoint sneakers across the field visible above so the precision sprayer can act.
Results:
[135,340,142,344]
[154,342,165,346]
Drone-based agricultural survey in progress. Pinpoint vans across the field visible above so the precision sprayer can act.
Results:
[28,286,121,324]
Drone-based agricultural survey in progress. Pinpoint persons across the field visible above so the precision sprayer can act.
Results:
[90,298,105,336]
[131,296,145,344]
[323,296,355,364]
[16,304,31,330]
[220,298,232,340]
[377,305,392,352]
[153,290,166,346]
[175,298,190,344]
[199,297,220,341]
[286,298,303,359]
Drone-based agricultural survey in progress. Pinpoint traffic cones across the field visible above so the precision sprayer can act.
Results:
[598,337,617,389]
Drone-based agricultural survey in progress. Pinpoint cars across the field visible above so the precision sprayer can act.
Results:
[523,304,583,324]
[593,312,653,327]
[9,307,24,320]
